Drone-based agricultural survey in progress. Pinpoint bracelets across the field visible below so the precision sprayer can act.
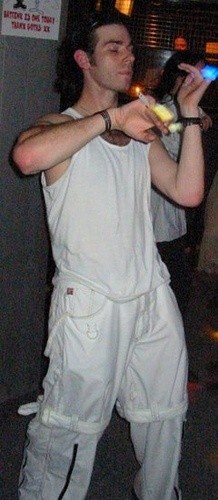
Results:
[99,110,112,134]
[182,117,203,129]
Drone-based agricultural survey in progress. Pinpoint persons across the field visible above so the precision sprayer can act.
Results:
[146,48,212,393]
[11,3,206,500]
